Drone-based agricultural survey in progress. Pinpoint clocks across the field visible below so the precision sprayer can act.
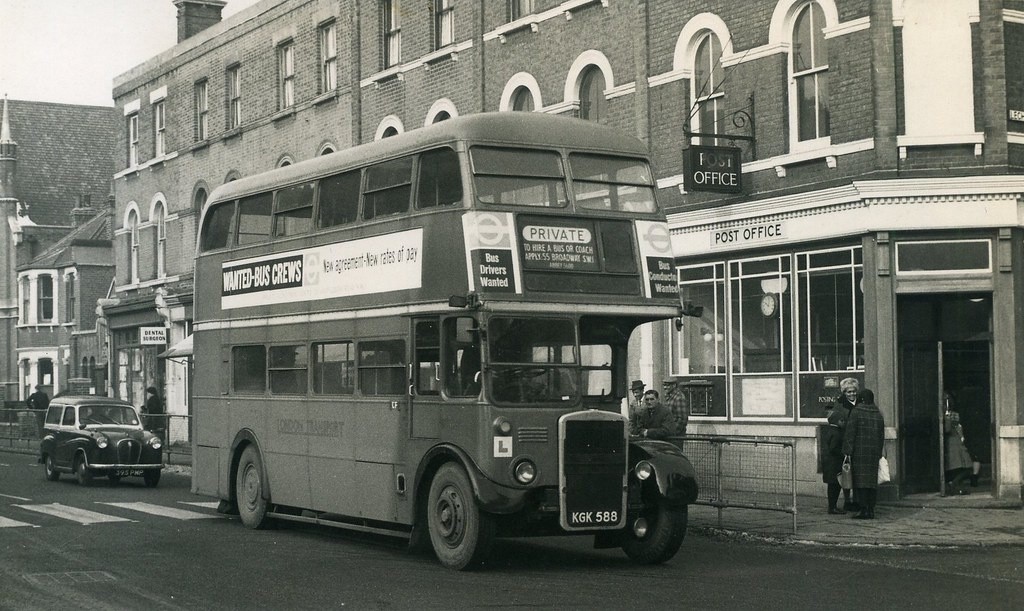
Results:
[760,292,778,318]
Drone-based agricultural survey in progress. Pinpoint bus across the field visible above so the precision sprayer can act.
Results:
[185,110,703,571]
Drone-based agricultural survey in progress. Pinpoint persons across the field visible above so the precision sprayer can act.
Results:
[460,318,526,398]
[146,387,165,435]
[827,378,868,514]
[842,389,884,519]
[629,378,688,453]
[27,386,49,438]
[933,391,972,495]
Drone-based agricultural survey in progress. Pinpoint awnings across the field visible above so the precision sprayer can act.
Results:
[157,334,193,364]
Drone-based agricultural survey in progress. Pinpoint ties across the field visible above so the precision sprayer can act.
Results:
[639,400,642,406]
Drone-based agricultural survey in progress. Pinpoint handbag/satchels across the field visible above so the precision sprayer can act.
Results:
[842,456,854,489]
[878,459,891,485]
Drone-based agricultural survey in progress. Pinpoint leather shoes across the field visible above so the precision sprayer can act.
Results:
[830,506,843,514]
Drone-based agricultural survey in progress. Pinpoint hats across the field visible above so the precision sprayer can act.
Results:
[662,377,677,383]
[628,380,646,390]
[147,387,157,394]
[824,411,842,424]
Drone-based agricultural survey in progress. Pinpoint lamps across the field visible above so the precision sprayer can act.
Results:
[712,329,724,342]
[699,328,712,342]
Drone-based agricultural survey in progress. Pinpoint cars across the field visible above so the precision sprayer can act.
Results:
[38,398,163,489]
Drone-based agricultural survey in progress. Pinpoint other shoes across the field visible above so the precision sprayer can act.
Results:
[852,508,874,519]
[958,490,971,496]
[843,500,858,511]
